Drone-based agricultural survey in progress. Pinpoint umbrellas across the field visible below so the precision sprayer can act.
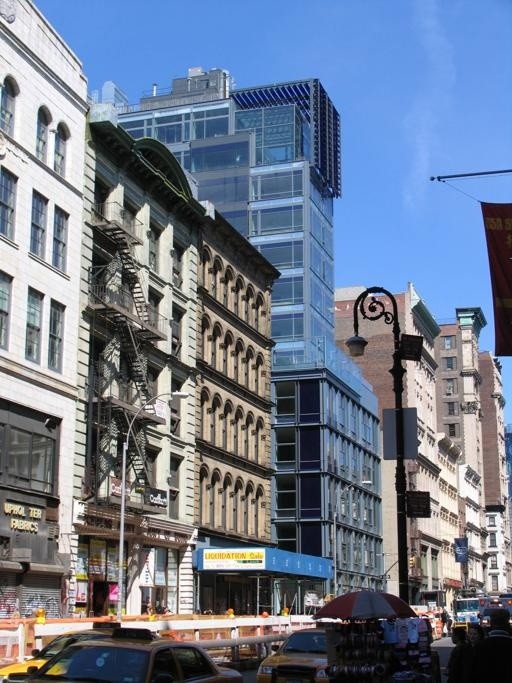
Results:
[311,589,418,618]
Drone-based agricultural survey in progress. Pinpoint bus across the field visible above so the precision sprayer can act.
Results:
[450,593,512,630]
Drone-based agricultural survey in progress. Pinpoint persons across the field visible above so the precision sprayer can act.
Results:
[468,623,485,646]
[447,627,472,682]
[463,608,511,682]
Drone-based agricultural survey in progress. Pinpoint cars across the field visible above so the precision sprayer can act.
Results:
[304,590,322,607]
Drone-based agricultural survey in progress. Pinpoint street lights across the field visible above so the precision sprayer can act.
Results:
[117,391,189,621]
[344,286,430,606]
[333,480,373,599]
